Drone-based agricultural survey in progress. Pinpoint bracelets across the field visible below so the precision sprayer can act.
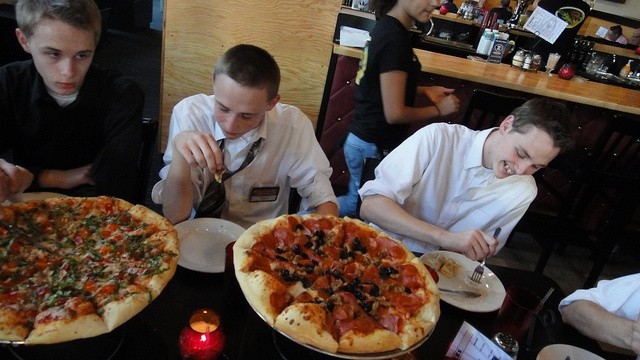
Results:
[433,104,441,117]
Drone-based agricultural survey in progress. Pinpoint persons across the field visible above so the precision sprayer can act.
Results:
[358,98,577,261]
[606,24,627,45]
[0,0,145,204]
[559,272,639,355]
[490,0,513,23]
[296,0,460,216]
[151,44,339,230]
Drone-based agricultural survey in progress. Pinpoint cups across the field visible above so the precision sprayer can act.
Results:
[488,33,514,64]
[224,241,238,284]
[493,285,545,340]
[586,55,603,75]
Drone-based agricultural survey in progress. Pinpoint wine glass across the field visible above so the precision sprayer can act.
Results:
[544,53,561,77]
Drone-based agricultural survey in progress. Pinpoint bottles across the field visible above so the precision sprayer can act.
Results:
[491,332,519,360]
[178,312,223,360]
[531,55,542,70]
[512,51,524,67]
[470,11,492,48]
[490,12,500,29]
[464,6,473,19]
[472,7,482,22]
[523,57,532,69]
[610,54,618,76]
[456,3,465,19]
[476,29,495,58]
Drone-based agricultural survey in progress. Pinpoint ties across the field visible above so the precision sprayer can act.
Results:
[194,137,264,219]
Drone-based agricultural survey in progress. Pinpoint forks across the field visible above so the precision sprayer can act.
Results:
[471,226,502,283]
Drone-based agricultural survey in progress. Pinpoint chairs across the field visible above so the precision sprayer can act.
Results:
[136,116,159,201]
[355,158,381,214]
[462,87,528,130]
[508,113,640,289]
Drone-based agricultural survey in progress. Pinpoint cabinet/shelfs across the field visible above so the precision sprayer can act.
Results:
[421,16,479,55]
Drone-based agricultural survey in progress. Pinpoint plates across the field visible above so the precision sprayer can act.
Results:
[175,218,246,272]
[536,343,605,360]
[420,251,506,312]
[555,7,585,28]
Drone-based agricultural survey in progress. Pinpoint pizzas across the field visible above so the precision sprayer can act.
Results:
[1,194,179,345]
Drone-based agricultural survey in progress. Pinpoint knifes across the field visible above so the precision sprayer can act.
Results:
[439,287,482,299]
